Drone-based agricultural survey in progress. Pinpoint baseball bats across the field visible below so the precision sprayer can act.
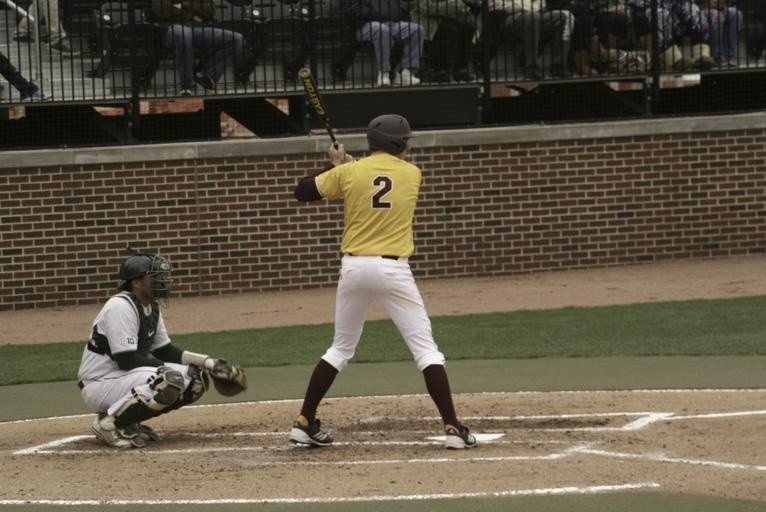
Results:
[298,68,338,151]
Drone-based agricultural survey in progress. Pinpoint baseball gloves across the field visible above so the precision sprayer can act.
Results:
[208,357,247,398]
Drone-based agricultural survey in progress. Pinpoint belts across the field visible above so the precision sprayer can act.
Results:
[381,252,399,261]
[76,380,86,390]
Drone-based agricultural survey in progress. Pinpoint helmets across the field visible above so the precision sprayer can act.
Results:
[122,256,172,310]
[368,115,419,155]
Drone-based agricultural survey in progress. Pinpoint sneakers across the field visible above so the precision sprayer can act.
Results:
[673,58,692,71]
[378,72,391,88]
[443,426,478,449]
[180,84,192,95]
[696,56,713,70]
[291,417,335,448]
[90,413,149,449]
[392,69,420,87]
[195,69,215,90]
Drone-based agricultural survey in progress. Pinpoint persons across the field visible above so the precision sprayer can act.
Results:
[288,112,477,448]
[1,1,743,101]
[77,255,247,450]
